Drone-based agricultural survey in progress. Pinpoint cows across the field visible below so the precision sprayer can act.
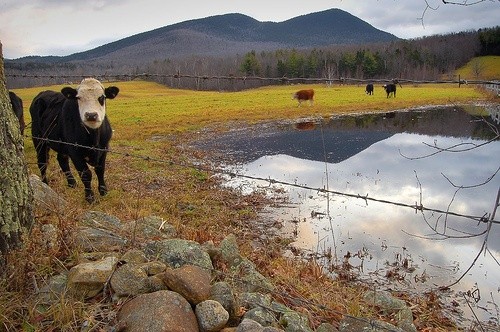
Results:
[291,88,315,109]
[29,77,120,205]
[382,83,397,99]
[365,83,374,95]
